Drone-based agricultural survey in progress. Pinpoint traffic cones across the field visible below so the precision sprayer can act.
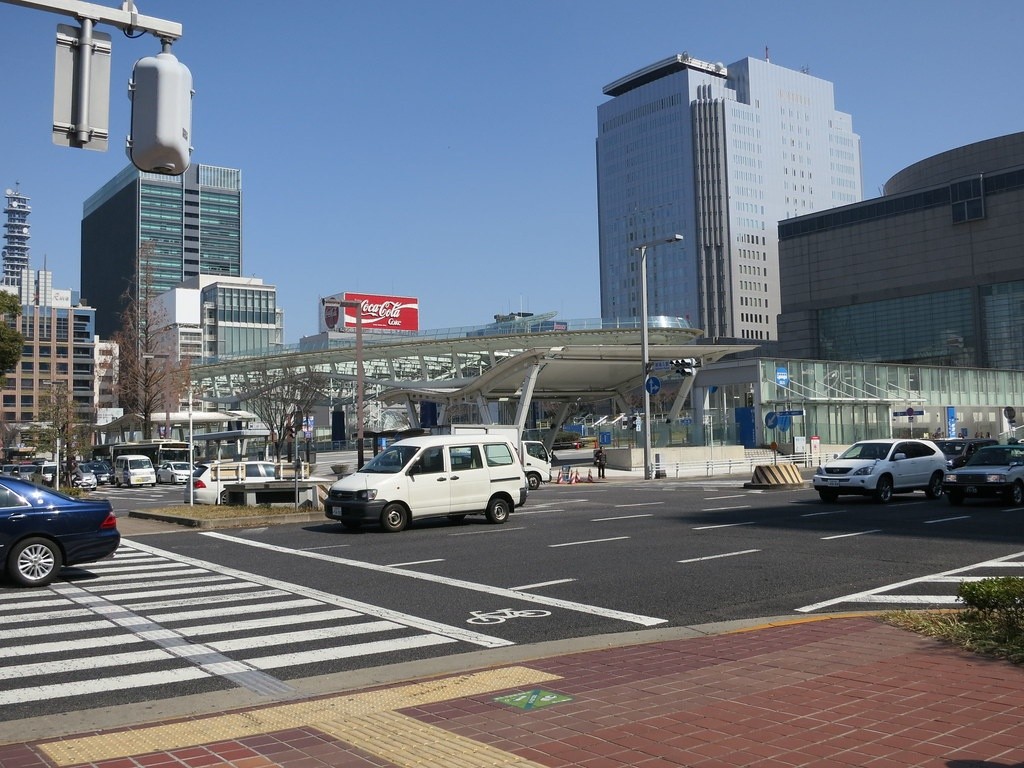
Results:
[587,468,594,482]
[570,469,581,484]
[557,468,564,484]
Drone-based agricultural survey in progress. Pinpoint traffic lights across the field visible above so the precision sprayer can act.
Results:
[670,358,697,376]
[628,416,637,430]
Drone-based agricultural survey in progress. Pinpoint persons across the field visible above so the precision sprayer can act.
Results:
[958,433,964,439]
[975,432,980,438]
[71,462,83,481]
[595,446,607,478]
[980,432,985,438]
[31,468,45,484]
[985,432,991,438]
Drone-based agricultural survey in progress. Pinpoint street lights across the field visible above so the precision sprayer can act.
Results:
[635,234,683,480]
[322,299,365,471]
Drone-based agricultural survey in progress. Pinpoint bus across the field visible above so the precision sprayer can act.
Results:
[92,439,194,476]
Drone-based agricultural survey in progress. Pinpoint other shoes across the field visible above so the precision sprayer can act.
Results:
[602,476,605,478]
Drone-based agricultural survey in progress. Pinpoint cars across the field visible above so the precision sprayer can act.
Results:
[0,476,120,588]
[813,438,1024,506]
[184,461,332,505]
[0,454,115,492]
[157,462,199,485]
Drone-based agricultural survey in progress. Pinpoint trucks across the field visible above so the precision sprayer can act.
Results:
[430,424,552,490]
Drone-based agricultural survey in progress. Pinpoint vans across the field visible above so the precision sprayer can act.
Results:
[115,455,156,488]
[324,435,528,532]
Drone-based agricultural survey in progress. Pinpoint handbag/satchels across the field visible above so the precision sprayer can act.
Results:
[594,459,601,467]
